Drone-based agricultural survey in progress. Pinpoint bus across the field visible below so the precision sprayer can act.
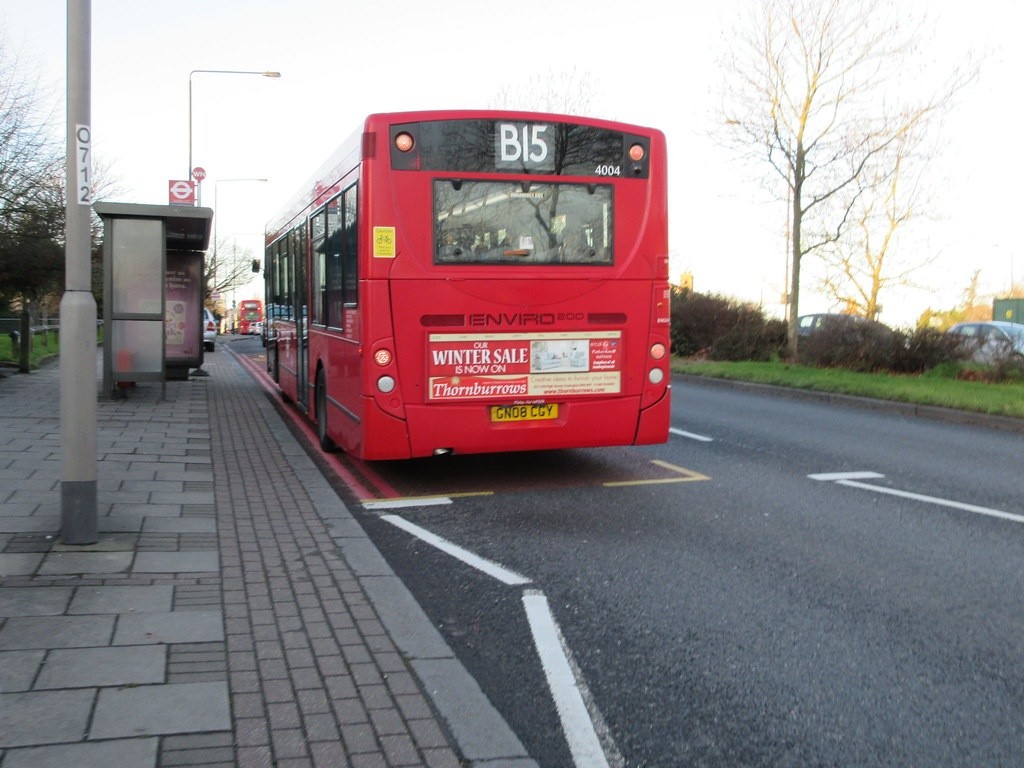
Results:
[234,300,262,334]
[251,109,672,459]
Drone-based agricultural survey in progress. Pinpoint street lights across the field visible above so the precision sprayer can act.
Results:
[189,70,281,183]
[214,179,268,308]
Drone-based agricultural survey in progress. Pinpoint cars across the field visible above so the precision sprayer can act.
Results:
[947,321,1024,365]
[254,321,264,334]
[248,323,255,333]
[201,307,218,352]
[796,313,871,339]
